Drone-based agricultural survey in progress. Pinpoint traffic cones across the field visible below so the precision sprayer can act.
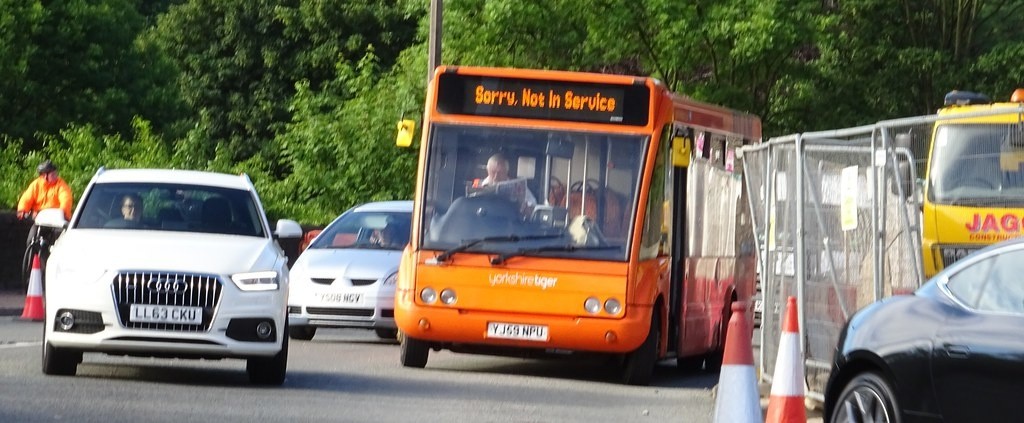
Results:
[766,294,809,422]
[16,255,46,322]
[710,299,765,423]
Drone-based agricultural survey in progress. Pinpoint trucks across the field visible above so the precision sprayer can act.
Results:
[907,90,1024,284]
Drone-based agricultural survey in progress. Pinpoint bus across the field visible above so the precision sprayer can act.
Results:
[396,64,763,384]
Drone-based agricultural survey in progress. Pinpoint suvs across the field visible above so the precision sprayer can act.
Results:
[41,165,303,383]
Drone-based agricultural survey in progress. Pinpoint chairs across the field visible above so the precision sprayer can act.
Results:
[549,176,622,239]
[200,196,232,225]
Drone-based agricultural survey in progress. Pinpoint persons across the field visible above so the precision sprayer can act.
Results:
[370,228,398,248]
[119,194,143,222]
[481,153,537,221]
[16,160,72,272]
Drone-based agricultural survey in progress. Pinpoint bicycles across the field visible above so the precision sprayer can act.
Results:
[20,210,56,289]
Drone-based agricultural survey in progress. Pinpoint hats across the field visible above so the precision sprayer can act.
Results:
[37,160,57,174]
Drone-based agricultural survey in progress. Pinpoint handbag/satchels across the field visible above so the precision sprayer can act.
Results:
[570,214,603,247]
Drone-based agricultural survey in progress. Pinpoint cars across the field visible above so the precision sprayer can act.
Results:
[289,200,414,339]
[822,236,1024,423]
